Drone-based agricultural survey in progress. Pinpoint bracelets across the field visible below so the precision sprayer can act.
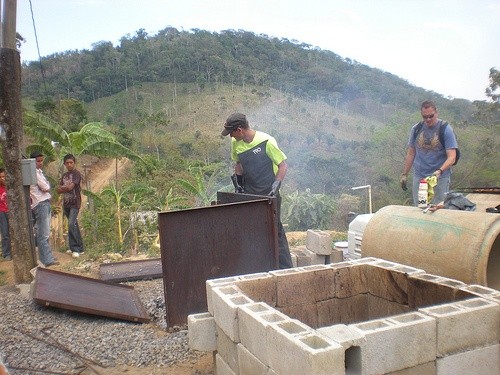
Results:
[436,168,443,175]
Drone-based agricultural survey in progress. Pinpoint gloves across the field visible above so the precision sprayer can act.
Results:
[431,169,442,182]
[267,179,282,197]
[230,174,245,193]
[399,172,408,191]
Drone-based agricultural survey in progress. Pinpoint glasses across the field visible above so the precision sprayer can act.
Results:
[422,112,435,119]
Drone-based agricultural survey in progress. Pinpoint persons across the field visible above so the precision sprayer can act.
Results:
[28,152,60,266]
[0,167,12,262]
[57,153,86,258]
[222,113,293,269]
[400,100,460,209]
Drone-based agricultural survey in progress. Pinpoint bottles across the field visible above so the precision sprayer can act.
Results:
[418,180,428,207]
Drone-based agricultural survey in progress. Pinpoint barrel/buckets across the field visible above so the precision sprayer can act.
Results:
[334,242,348,262]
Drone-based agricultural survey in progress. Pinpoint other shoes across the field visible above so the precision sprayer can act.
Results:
[47,260,60,266]
[66,249,73,253]
[5,255,13,261]
[71,251,80,258]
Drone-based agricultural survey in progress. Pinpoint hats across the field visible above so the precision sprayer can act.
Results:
[29,152,45,158]
[221,112,247,136]
